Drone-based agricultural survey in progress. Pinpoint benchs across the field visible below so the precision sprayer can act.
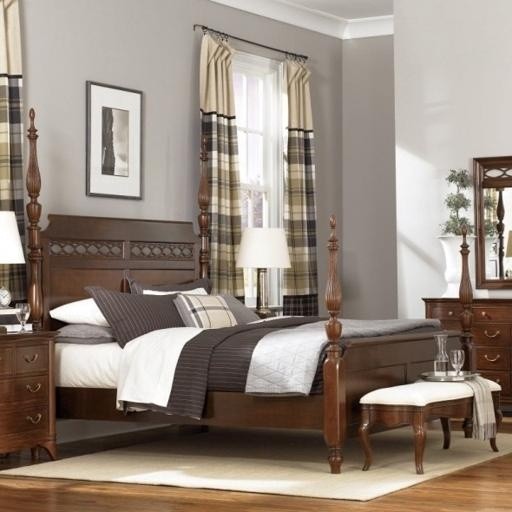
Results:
[359,376,501,474]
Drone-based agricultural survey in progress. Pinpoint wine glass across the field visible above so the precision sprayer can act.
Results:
[451,349,465,376]
[14,303,32,333]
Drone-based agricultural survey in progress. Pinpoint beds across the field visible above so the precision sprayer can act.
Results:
[26,106,477,474]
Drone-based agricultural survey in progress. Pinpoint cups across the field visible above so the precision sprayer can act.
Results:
[434,334,449,375]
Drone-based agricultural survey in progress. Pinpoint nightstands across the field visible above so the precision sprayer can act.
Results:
[0,331,60,461]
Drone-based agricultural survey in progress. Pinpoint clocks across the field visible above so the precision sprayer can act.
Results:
[0,287,12,308]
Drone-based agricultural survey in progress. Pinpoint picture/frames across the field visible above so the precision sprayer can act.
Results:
[85,80,143,200]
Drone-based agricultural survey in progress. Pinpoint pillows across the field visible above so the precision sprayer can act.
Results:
[216,292,260,324]
[84,286,185,349]
[48,298,111,328]
[128,277,212,295]
[141,288,208,295]
[52,324,117,344]
[172,294,239,328]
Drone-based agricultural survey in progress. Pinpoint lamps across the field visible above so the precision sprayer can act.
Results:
[0,211,26,265]
[236,227,292,319]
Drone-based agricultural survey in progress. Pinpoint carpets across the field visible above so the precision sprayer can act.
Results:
[0,429,512,502]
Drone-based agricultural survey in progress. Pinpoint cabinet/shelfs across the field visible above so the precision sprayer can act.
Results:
[422,297,512,413]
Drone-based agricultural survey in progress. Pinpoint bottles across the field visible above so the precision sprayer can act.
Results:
[432,334,449,375]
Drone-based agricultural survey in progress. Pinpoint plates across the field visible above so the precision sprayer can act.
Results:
[420,370,479,382]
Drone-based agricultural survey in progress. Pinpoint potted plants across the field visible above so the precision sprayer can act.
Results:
[437,169,477,298]
[484,195,499,280]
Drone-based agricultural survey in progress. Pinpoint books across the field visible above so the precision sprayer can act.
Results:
[0,324,33,333]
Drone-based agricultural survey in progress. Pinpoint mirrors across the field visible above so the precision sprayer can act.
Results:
[473,155,512,289]
